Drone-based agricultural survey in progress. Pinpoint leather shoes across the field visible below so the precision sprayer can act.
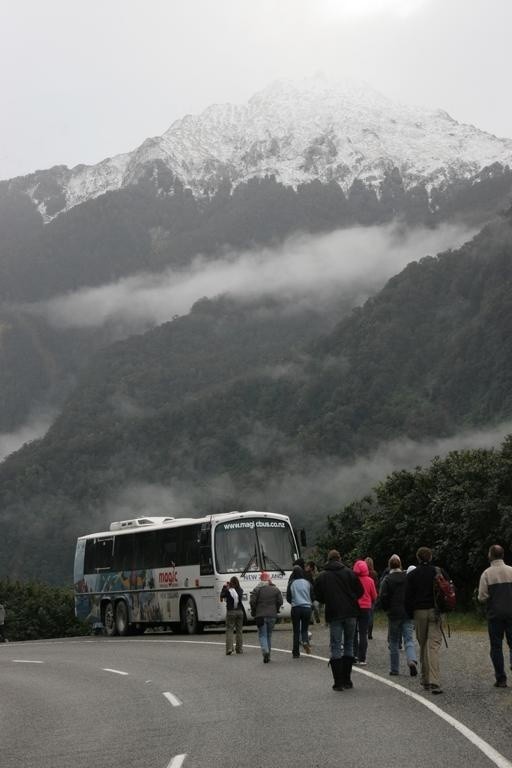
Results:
[263,652,272,664]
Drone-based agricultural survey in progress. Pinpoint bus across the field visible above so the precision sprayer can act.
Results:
[73,506,313,632]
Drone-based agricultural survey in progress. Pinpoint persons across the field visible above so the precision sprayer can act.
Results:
[349,560,378,666]
[291,557,314,587]
[405,545,456,695]
[471,544,512,689]
[249,571,283,664]
[304,560,321,625]
[313,549,365,693]
[378,553,418,678]
[362,556,379,640]
[0,602,11,646]
[285,565,316,659]
[220,576,245,656]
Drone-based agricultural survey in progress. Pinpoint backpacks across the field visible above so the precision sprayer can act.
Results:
[432,566,456,616]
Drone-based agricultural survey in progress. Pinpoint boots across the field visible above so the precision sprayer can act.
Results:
[343,657,353,689]
[330,658,343,690]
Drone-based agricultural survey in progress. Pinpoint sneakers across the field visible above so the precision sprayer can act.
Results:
[353,656,368,666]
[226,648,243,656]
[302,642,312,656]
[390,672,399,676]
[493,680,507,687]
[408,661,418,677]
[293,653,300,658]
[421,684,444,693]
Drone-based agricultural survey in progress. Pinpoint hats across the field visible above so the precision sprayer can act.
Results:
[260,571,273,585]
[406,564,417,575]
[389,553,401,569]
